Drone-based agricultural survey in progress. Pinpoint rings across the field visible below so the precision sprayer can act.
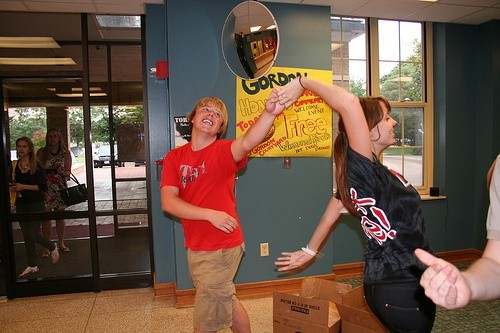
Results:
[281,94,284,99]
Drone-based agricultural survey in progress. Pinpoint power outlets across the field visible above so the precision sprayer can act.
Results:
[259,242,269,257]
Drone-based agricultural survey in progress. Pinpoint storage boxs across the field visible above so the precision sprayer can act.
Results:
[272,276,352,333]
[335,285,387,333]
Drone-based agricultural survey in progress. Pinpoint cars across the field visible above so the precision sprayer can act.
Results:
[94,142,125,168]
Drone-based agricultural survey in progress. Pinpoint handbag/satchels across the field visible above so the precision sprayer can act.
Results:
[10,160,18,207]
[60,171,88,207]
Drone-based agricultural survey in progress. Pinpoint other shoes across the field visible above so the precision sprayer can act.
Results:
[60,247,71,252]
[42,249,51,258]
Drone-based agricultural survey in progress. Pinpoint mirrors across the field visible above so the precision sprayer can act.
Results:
[221,1,280,81]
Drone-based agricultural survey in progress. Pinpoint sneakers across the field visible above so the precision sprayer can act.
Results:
[51,243,59,263]
[19,266,39,277]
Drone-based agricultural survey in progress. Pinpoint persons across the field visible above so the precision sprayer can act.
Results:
[413,152,499,310]
[9,136,60,278]
[159,86,285,333]
[36,126,72,259]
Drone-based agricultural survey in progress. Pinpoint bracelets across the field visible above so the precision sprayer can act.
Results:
[298,76,305,89]
[274,76,437,333]
[300,244,317,258]
